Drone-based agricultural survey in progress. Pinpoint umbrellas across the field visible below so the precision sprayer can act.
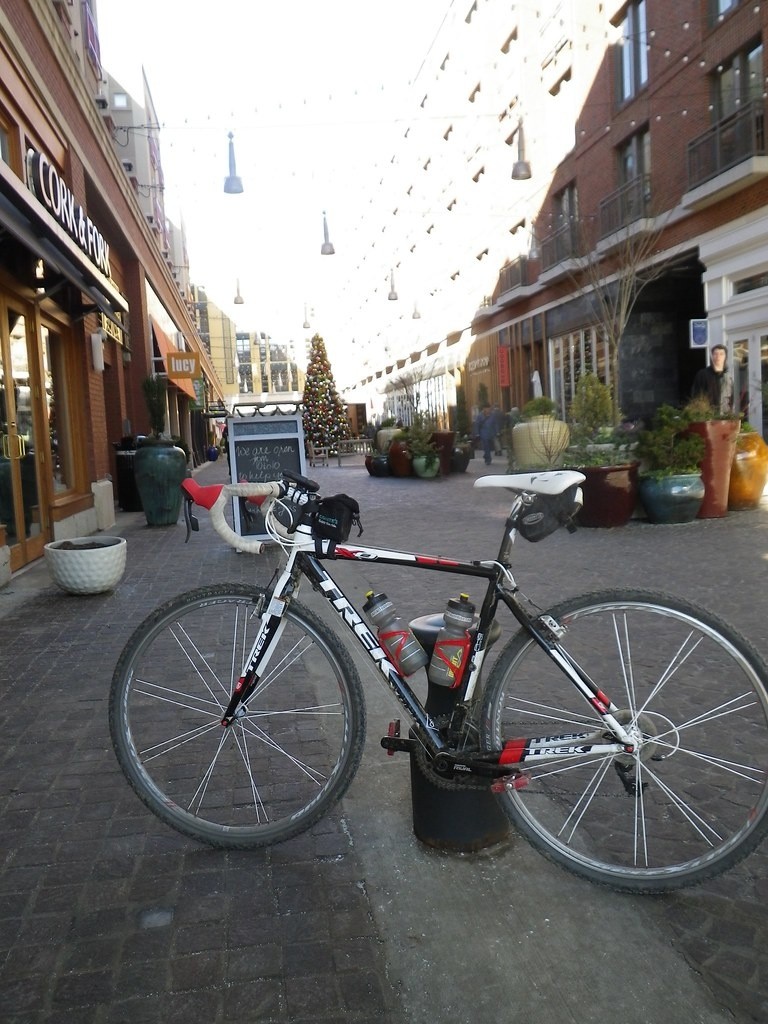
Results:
[532,370,542,397]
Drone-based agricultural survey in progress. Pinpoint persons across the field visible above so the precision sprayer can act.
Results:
[396,417,403,429]
[220,415,235,475]
[692,344,735,415]
[475,403,519,465]
[366,422,375,447]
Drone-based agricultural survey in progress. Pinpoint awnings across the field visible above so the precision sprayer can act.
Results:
[152,321,197,401]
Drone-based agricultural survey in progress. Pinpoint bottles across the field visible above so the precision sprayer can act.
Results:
[430,593,475,688]
[362,590,429,677]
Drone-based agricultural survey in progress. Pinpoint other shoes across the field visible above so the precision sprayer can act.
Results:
[483,453,492,465]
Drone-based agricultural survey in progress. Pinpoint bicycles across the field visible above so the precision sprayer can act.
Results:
[108,469,767,895]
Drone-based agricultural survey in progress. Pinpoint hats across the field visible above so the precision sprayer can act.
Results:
[483,402,492,407]
